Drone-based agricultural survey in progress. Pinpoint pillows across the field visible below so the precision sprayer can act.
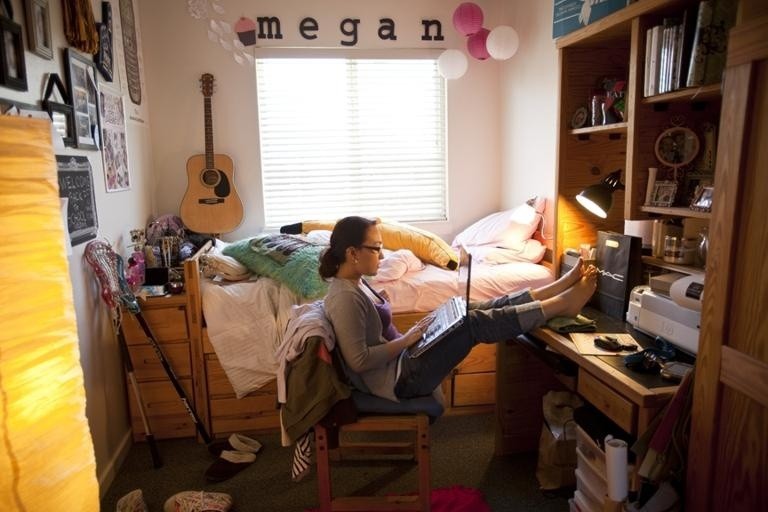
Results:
[451,195,547,248]
[465,239,546,264]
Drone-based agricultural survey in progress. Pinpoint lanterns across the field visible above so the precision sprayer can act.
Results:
[466,28,492,60]
[454,2,483,37]
[486,26,519,62]
[438,49,467,80]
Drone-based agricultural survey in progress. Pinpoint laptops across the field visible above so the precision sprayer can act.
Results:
[408,243,472,360]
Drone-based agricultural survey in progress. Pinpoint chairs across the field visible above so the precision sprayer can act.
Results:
[316,410,432,512]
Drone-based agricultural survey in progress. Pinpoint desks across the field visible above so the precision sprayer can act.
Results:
[495,301,688,492]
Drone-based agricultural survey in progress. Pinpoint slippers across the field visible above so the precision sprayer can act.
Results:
[210,433,263,453]
[205,450,256,485]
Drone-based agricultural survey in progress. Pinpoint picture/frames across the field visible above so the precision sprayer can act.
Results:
[24,0,54,61]
[46,99,76,147]
[99,81,132,194]
[96,22,116,80]
[66,46,103,152]
[55,155,100,246]
[0,17,29,91]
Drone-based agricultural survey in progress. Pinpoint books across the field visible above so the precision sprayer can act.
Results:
[643,3,729,96]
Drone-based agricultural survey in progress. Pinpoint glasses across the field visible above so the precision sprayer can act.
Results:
[358,244,383,253]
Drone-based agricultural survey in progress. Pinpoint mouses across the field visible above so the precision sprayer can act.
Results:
[595,335,622,354]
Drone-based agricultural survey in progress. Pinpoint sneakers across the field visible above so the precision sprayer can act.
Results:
[114,488,145,511]
[163,491,233,512]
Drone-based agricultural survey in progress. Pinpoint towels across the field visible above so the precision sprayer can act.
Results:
[543,314,598,331]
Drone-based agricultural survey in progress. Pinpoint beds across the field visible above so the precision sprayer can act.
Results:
[184,197,554,442]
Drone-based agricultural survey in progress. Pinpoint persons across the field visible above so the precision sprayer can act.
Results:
[319,216,598,402]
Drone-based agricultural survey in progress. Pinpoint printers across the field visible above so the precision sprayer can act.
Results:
[625,274,705,358]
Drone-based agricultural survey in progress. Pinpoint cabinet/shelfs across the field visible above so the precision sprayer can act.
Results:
[116,293,197,444]
[553,0,736,283]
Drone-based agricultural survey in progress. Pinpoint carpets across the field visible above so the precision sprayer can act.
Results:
[302,484,490,512]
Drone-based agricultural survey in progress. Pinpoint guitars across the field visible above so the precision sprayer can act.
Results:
[179,72,244,233]
